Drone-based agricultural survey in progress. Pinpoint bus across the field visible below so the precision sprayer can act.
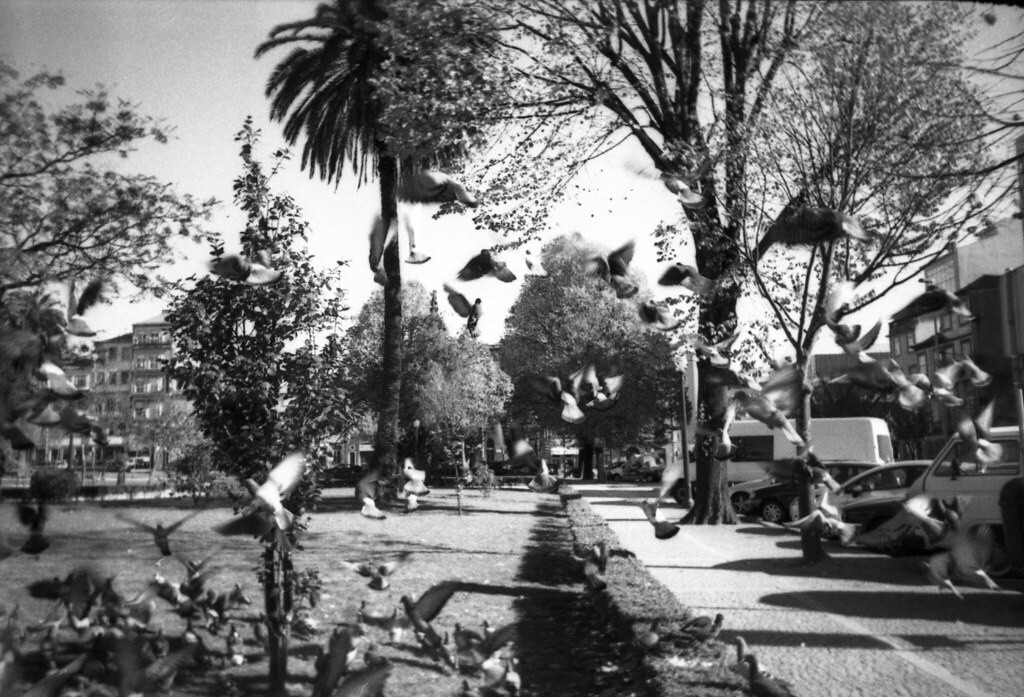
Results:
[658,414,896,504]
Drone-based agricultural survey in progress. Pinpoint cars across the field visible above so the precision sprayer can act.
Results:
[720,456,978,551]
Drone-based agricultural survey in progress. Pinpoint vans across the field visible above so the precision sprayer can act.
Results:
[906,423,1024,579]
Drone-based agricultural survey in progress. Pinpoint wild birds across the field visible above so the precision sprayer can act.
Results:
[392,156,480,208]
[0,209,1024,697]
